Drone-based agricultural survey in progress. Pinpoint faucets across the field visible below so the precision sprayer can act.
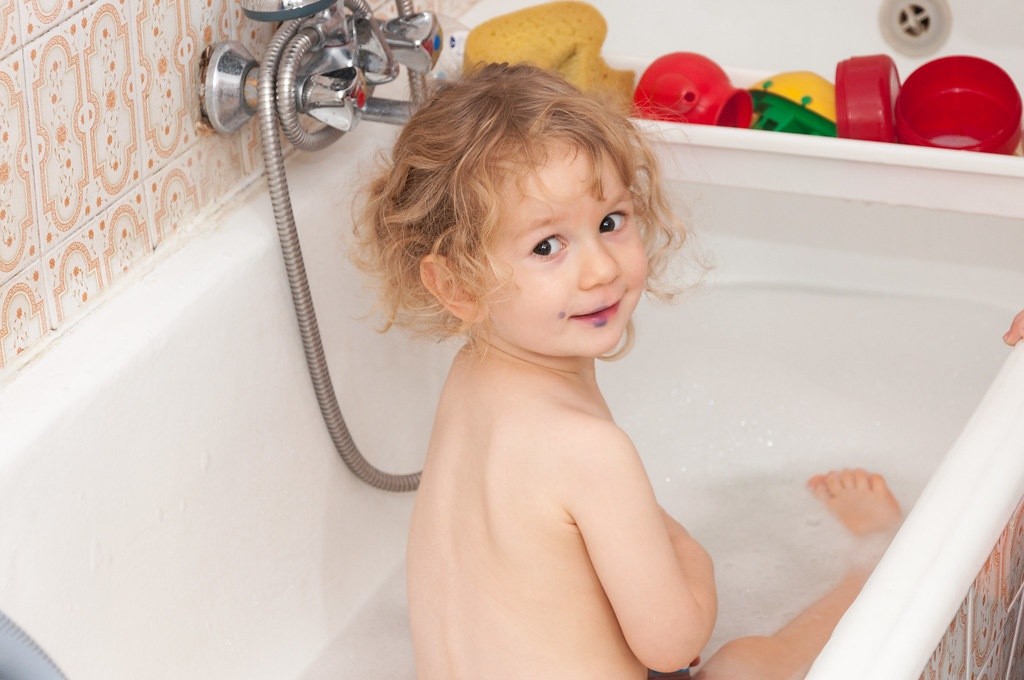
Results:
[358,97,420,127]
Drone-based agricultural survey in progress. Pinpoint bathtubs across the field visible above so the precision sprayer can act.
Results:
[0,0,1023,680]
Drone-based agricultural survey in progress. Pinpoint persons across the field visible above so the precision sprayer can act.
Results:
[1003,309,1024,346]
[348,61,906,680]
